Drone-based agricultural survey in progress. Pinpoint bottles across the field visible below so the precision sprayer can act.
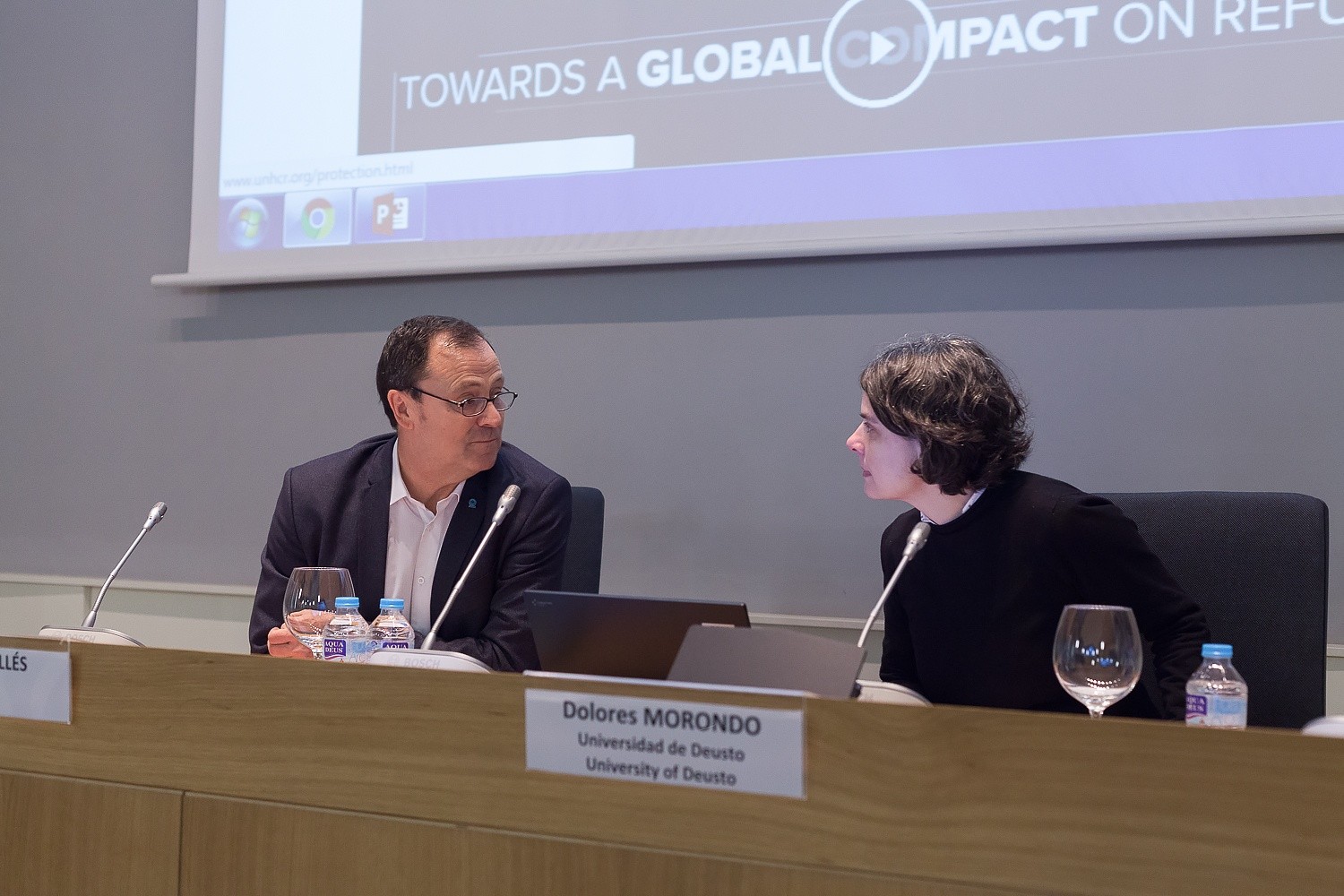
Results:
[369,598,415,651]
[1185,643,1248,729]
[322,596,372,664]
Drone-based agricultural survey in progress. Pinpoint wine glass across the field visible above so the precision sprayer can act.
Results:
[1052,604,1142,719]
[282,567,355,662]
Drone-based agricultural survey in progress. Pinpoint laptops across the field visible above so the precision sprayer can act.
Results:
[523,590,751,682]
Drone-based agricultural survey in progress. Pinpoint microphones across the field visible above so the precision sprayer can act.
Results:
[856,521,931,704]
[38,502,169,648]
[369,484,522,672]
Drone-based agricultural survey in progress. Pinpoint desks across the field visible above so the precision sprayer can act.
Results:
[0,636,1343,896]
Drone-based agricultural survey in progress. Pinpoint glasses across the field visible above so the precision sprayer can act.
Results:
[407,387,517,418]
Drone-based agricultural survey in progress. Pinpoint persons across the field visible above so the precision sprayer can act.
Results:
[248,315,573,673]
[846,334,1213,722]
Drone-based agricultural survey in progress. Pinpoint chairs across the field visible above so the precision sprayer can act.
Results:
[1091,491,1327,729]
[556,486,605,595]
